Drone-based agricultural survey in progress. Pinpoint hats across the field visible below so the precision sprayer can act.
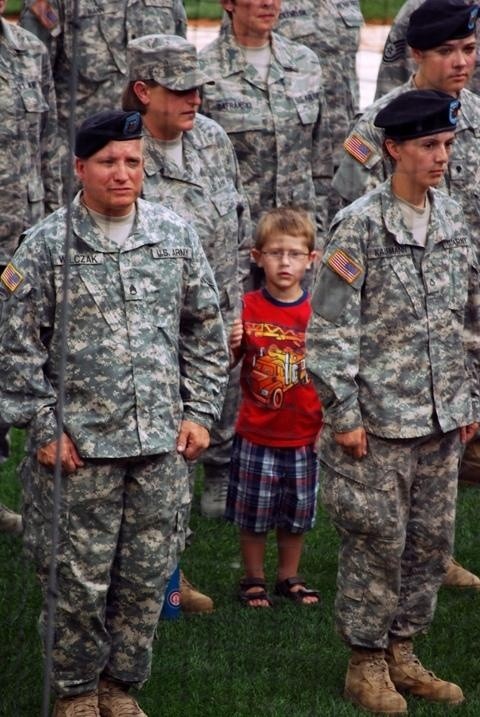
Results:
[126,33,215,92]
[408,0,479,50]
[373,90,461,141]
[75,111,142,161]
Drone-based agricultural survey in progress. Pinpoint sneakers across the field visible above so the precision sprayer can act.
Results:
[387,644,465,706]
[51,688,100,717]
[0,501,23,533]
[179,568,213,615]
[343,649,408,716]
[457,444,480,485]
[200,473,230,519]
[99,678,147,716]
[440,558,480,591]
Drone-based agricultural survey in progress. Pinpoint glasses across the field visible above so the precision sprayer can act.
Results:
[261,248,309,261]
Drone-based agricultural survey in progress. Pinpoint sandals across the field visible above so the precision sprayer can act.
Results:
[271,576,324,607]
[236,575,274,609]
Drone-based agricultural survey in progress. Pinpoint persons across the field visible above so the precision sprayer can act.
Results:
[329,0,480,593]
[213,0,368,192]
[373,0,480,483]
[18,0,194,154]
[1,2,63,534]
[303,89,480,714]
[217,204,341,610]
[0,108,232,717]
[194,0,340,520]
[66,35,252,614]
